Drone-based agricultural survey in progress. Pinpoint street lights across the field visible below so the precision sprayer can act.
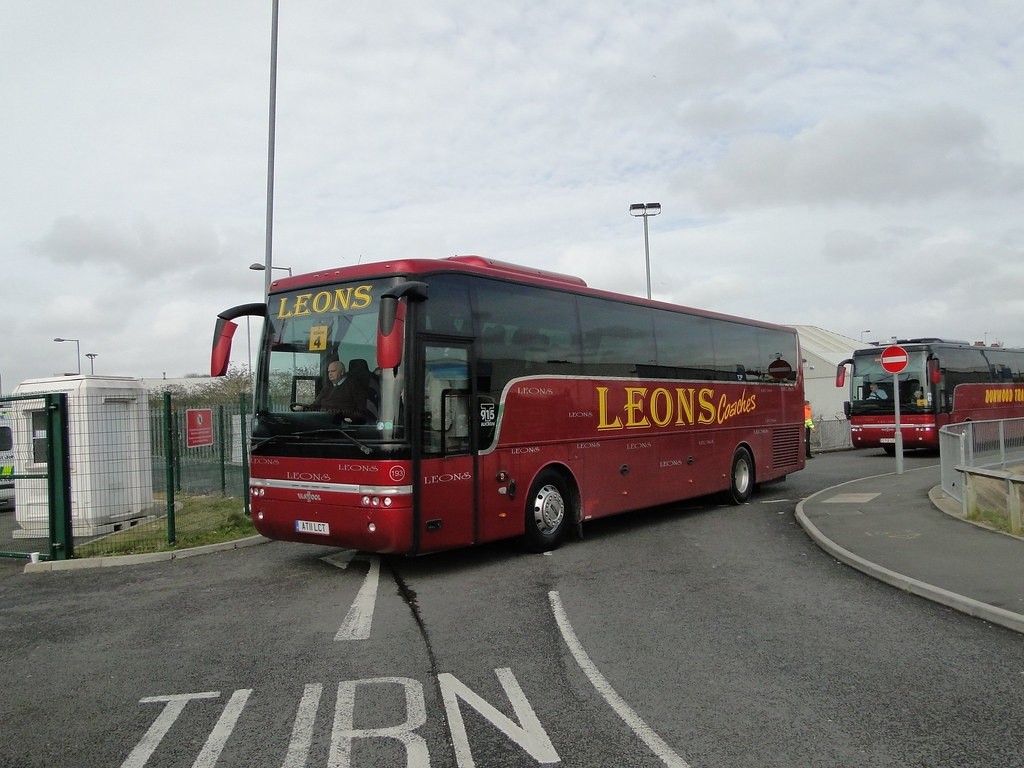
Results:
[629,202,661,302]
[85,353,98,375]
[248,263,296,375]
[53,337,80,374]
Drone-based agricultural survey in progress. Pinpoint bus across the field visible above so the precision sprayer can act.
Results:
[210,254,806,556]
[836,338,1024,457]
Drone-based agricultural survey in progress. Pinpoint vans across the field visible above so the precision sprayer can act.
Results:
[0,408,15,510]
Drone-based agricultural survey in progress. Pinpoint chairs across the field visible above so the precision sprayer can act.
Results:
[346,359,378,412]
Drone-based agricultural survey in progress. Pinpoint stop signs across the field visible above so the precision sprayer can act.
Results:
[881,345,909,374]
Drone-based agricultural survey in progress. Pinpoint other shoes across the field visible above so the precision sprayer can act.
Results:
[807,455,815,458]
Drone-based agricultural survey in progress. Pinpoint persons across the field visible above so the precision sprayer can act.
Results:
[293,361,351,412]
[911,391,923,403]
[866,384,888,400]
[805,401,816,458]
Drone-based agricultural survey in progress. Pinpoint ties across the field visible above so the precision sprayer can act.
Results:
[335,382,338,386]
[874,392,877,396]
